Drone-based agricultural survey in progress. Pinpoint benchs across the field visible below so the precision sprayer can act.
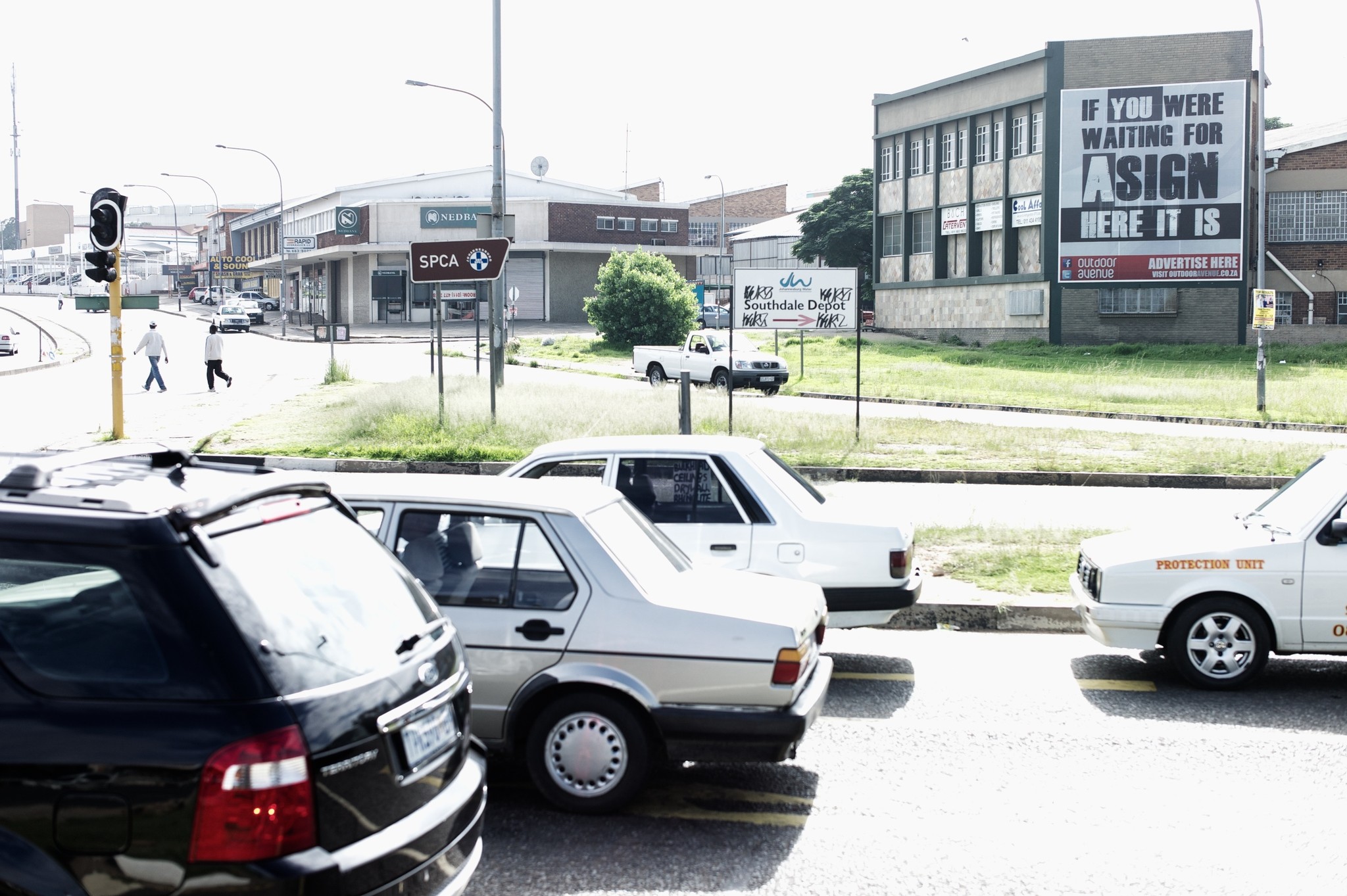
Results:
[403,530,452,606]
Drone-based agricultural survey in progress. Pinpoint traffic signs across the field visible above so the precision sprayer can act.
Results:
[733,267,858,331]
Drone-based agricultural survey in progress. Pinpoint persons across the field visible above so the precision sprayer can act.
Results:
[133,321,168,393]
[105,283,110,293]
[599,464,631,491]
[399,512,453,580]
[27,280,32,294]
[58,292,63,310]
[204,325,232,391]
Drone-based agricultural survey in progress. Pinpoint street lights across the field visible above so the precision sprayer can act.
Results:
[33,199,72,297]
[79,191,129,297]
[404,80,509,351]
[123,184,182,313]
[704,174,724,331]
[160,173,223,307]
[214,144,286,338]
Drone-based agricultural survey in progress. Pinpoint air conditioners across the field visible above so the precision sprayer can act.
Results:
[651,238,667,246]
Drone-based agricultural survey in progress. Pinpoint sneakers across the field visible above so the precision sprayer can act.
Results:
[227,377,232,387]
[207,387,216,392]
[157,389,167,393]
[142,385,148,391]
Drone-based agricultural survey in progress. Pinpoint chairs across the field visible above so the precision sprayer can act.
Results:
[635,474,657,520]
[695,342,705,352]
[445,521,484,606]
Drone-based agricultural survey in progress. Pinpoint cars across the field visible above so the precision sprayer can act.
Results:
[859,309,874,323]
[0,325,20,356]
[292,468,833,813]
[222,298,245,306]
[237,299,265,324]
[0,271,142,287]
[357,435,926,630]
[230,291,280,311]
[1069,447,1347,691]
[692,304,730,331]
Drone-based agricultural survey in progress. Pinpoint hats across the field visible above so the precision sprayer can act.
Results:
[149,320,157,326]
[60,291,62,293]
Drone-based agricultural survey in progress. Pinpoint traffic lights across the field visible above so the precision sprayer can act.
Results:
[90,199,123,251]
[85,252,116,282]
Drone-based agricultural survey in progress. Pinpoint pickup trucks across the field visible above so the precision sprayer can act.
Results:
[212,305,250,334]
[632,330,789,396]
[193,291,207,305]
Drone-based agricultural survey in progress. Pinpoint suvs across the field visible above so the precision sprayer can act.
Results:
[204,286,242,305]
[0,432,491,896]
[189,287,209,302]
